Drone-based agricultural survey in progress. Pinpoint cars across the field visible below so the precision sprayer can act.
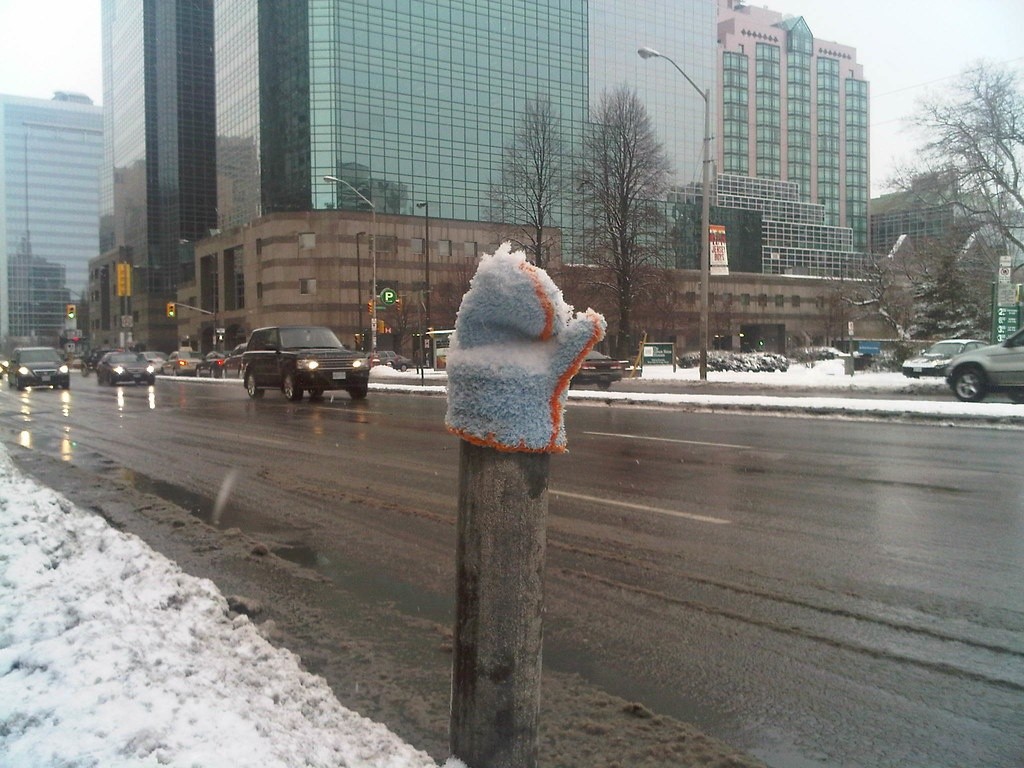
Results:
[8,347,70,389]
[96,352,155,386]
[367,349,413,374]
[940,330,1023,403]
[81,348,124,377]
[221,342,247,380]
[568,349,623,391]
[196,351,228,379]
[138,351,169,374]
[901,339,990,379]
[162,347,208,377]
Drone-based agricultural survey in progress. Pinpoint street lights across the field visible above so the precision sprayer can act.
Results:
[324,175,377,353]
[416,202,433,369]
[357,232,367,351]
[638,47,708,381]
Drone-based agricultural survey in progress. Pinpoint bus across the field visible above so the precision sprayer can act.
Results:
[412,329,454,369]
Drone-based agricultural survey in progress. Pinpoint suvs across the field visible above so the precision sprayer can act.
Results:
[239,325,372,401]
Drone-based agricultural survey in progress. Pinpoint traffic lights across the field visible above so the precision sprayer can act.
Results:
[395,298,401,312]
[355,333,361,345]
[167,304,177,320]
[367,301,374,314]
[67,305,77,321]
